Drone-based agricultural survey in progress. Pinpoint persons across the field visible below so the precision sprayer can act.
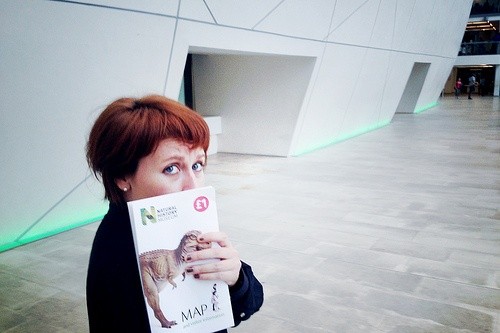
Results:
[454,78,462,97]
[87,95,263,333]
[459,39,474,55]
[467,76,478,99]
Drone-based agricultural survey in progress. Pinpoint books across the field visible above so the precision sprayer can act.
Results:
[126,187,235,333]
